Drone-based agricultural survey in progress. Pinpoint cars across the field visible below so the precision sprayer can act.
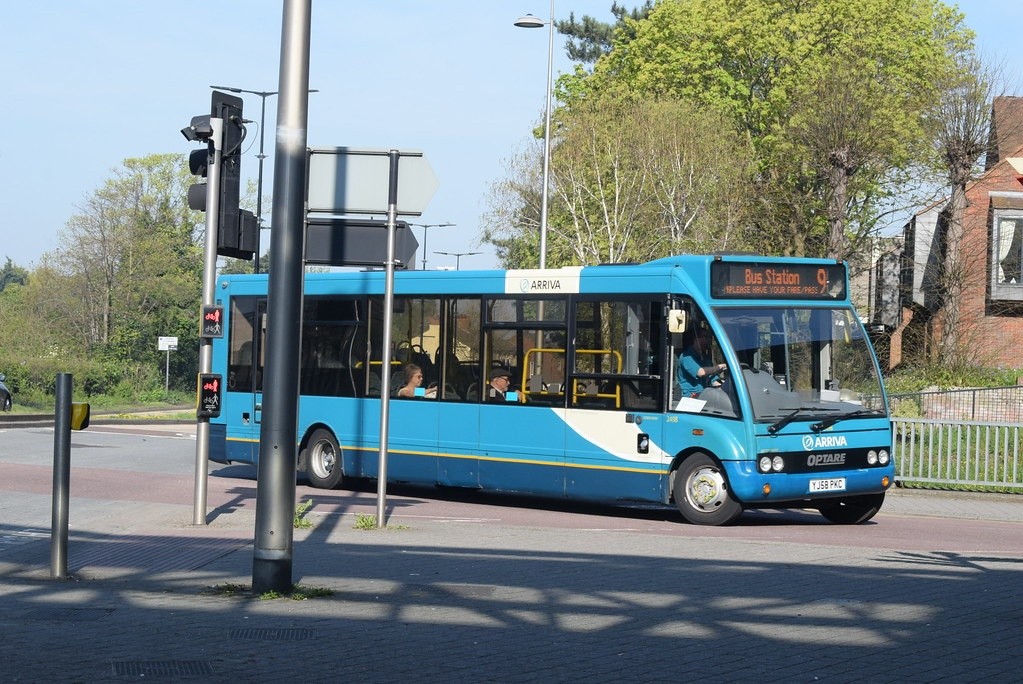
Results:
[0,372,13,414]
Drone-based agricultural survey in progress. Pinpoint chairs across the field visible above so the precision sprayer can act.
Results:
[363,341,504,400]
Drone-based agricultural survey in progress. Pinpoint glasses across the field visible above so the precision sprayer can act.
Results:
[414,374,423,379]
[502,378,512,384]
[697,335,710,339]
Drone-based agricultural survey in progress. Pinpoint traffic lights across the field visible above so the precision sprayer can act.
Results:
[188,90,256,261]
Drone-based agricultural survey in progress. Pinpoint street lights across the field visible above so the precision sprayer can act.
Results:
[408,221,456,354]
[433,251,484,354]
[209,83,320,275]
[510,0,557,376]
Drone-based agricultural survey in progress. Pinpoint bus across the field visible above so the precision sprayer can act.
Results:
[210,250,896,526]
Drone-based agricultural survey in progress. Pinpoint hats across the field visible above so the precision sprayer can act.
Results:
[490,369,513,378]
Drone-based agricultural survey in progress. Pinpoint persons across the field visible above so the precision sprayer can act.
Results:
[395,363,438,399]
[676,327,729,401]
[484,369,521,403]
